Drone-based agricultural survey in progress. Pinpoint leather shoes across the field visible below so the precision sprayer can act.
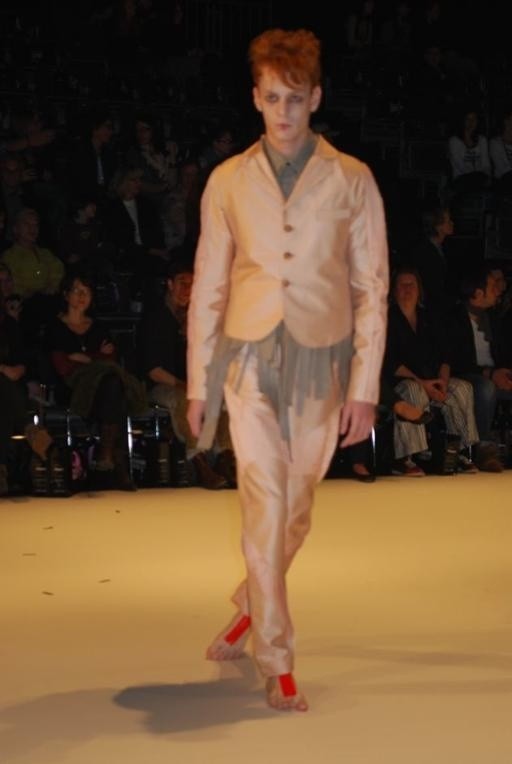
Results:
[396,405,433,424]
[0,447,238,498]
[350,446,505,482]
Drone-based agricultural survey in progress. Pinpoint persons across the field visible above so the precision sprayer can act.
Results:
[313,4,509,477]
[2,2,236,495]
[186,30,389,711]
